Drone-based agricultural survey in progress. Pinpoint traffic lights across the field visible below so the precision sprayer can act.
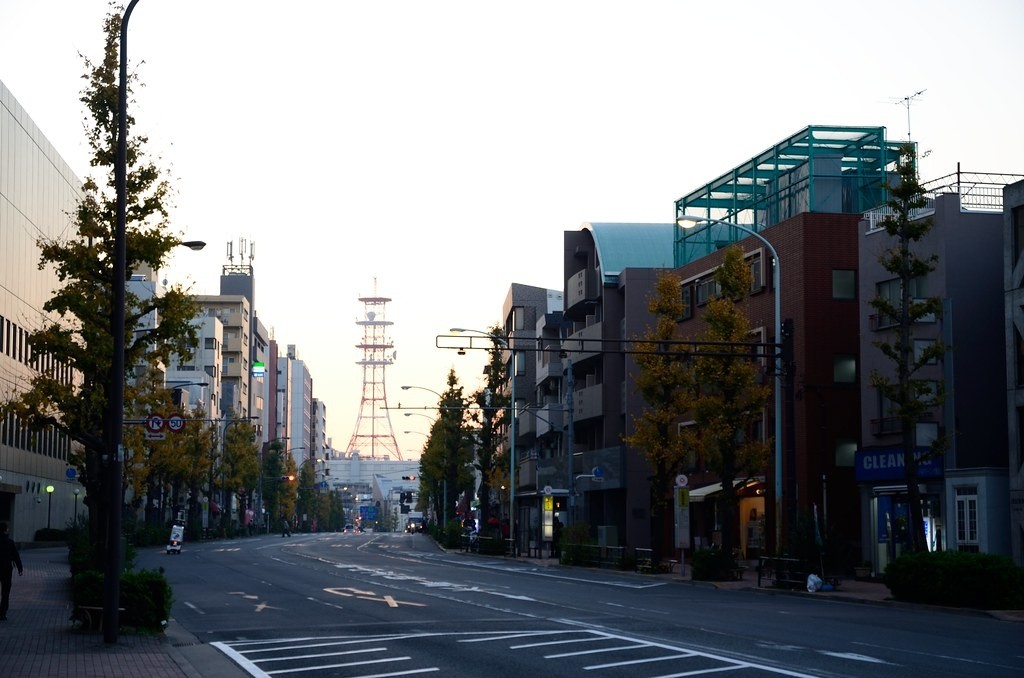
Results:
[401,475,415,481]
[281,475,295,481]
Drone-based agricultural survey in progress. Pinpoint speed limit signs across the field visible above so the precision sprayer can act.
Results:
[167,413,186,433]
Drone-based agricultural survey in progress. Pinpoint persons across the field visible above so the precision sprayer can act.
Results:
[488,514,499,537]
[282,521,291,537]
[0,523,23,619]
[549,517,564,558]
[247,520,254,536]
[501,514,510,538]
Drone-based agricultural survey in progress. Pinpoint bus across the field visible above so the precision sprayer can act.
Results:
[345,524,355,533]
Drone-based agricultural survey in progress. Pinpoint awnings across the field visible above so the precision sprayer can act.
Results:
[689,478,757,500]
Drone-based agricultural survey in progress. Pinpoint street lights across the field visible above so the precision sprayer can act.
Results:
[281,447,305,455]
[673,215,786,556]
[399,385,450,407]
[299,459,322,482]
[73,488,80,521]
[172,382,209,387]
[220,416,258,531]
[46,486,54,528]
[259,437,290,533]
[403,413,439,423]
[449,327,516,556]
[403,430,431,438]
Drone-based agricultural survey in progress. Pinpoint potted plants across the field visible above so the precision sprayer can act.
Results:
[855,559,871,578]
